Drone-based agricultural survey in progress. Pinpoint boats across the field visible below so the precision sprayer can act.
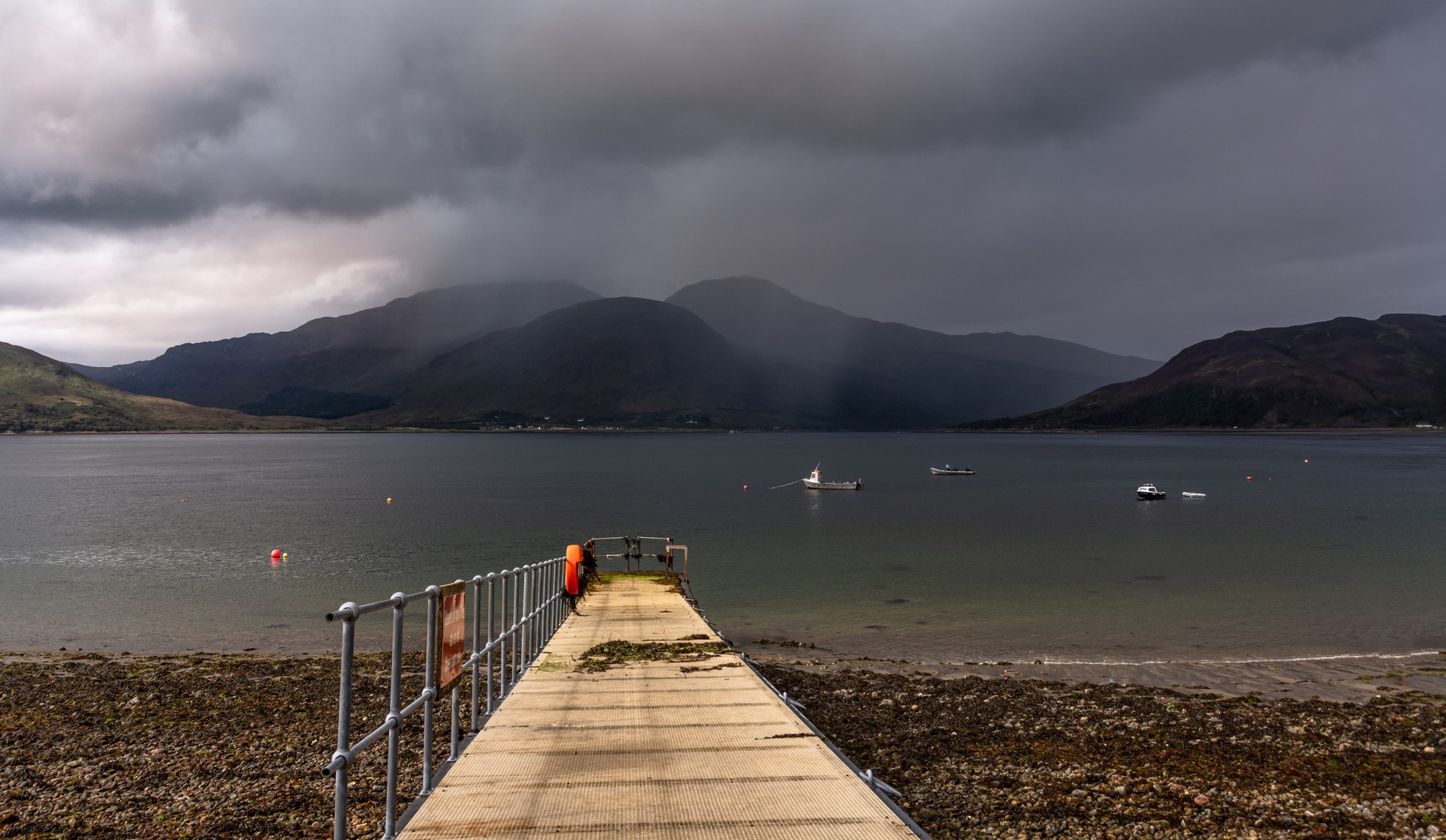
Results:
[1182,492,1206,497]
[1136,484,1166,499]
[930,464,976,475]
[802,462,864,490]
[1415,424,1437,431]
